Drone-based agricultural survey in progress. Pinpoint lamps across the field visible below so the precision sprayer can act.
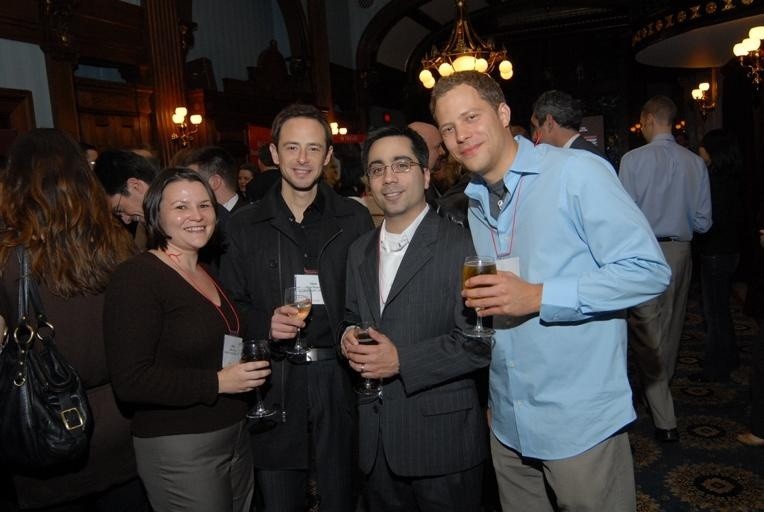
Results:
[732,25,764,91]
[168,107,201,149]
[418,4,513,90]
[691,82,716,118]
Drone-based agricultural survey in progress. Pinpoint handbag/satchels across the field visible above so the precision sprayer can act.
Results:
[0,240,93,479]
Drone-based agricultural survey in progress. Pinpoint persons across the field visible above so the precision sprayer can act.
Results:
[429,70,672,510]
[76,121,764,381]
[1,125,155,511]
[331,124,498,511]
[617,94,712,446]
[530,91,606,159]
[102,165,271,511]
[219,101,375,512]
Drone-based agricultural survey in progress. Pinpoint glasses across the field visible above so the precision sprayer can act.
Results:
[366,157,422,178]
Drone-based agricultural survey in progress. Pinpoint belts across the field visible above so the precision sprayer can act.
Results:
[656,237,671,242]
[291,347,337,364]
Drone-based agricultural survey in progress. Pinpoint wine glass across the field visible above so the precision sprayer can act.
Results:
[284,286,313,355]
[352,321,383,395]
[461,254,497,337]
[243,339,277,420]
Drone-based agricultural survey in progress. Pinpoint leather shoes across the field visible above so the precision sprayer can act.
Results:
[735,433,764,446]
[654,428,679,442]
